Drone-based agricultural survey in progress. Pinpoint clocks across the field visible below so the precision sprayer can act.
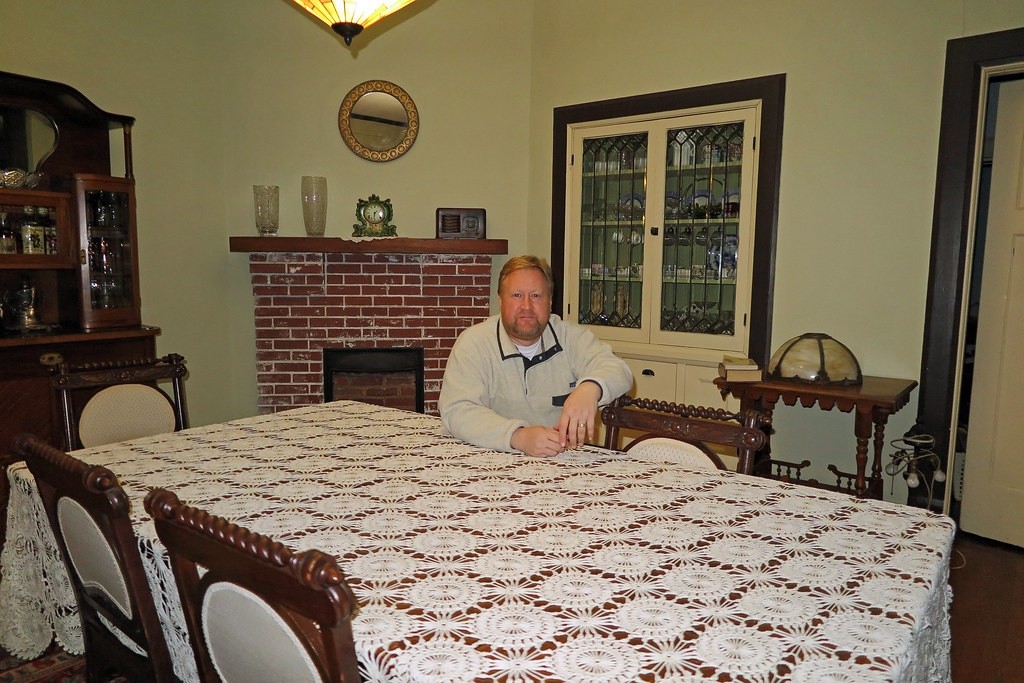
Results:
[352,193,397,236]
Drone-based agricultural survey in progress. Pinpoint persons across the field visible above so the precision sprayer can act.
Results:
[436,256,634,458]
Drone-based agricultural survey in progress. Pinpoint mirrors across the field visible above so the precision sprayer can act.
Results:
[337,80,420,162]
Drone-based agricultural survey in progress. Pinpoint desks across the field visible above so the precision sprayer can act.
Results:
[0,400,956,683]
[712,364,918,500]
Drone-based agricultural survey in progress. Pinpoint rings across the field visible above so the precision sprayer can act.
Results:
[578,423,586,427]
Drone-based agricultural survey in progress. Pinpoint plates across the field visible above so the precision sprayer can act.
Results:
[708,235,738,277]
[619,190,740,220]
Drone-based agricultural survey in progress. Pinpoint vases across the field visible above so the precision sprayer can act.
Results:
[301,175,328,236]
[253,184,279,235]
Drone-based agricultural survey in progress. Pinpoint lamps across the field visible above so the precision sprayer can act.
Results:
[884,433,966,570]
[293,0,416,46]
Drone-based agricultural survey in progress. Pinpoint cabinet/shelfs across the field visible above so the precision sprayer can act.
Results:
[563,98,762,424]
[0,72,161,550]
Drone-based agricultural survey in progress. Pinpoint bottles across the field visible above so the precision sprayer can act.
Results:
[88,236,112,275]
[0,212,18,255]
[90,278,121,310]
[16,205,46,255]
[85,190,121,229]
[37,207,58,255]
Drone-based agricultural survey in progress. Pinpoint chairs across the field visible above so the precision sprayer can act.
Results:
[601,394,773,476]
[12,432,185,683]
[48,353,190,452]
[142,486,365,683]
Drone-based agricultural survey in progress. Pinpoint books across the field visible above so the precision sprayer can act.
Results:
[718,351,762,382]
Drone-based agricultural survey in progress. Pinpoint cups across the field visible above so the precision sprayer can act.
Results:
[253,184,280,235]
[612,228,726,246]
[301,175,328,235]
